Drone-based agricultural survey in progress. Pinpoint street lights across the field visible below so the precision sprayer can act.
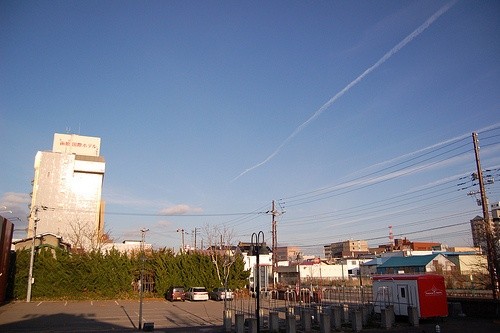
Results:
[138,229,150,331]
[246,230,270,333]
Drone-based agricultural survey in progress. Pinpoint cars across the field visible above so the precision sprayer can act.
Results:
[185,287,209,301]
[210,288,234,301]
[166,286,185,302]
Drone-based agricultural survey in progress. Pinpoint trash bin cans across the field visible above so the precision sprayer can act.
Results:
[142,322,155,332]
